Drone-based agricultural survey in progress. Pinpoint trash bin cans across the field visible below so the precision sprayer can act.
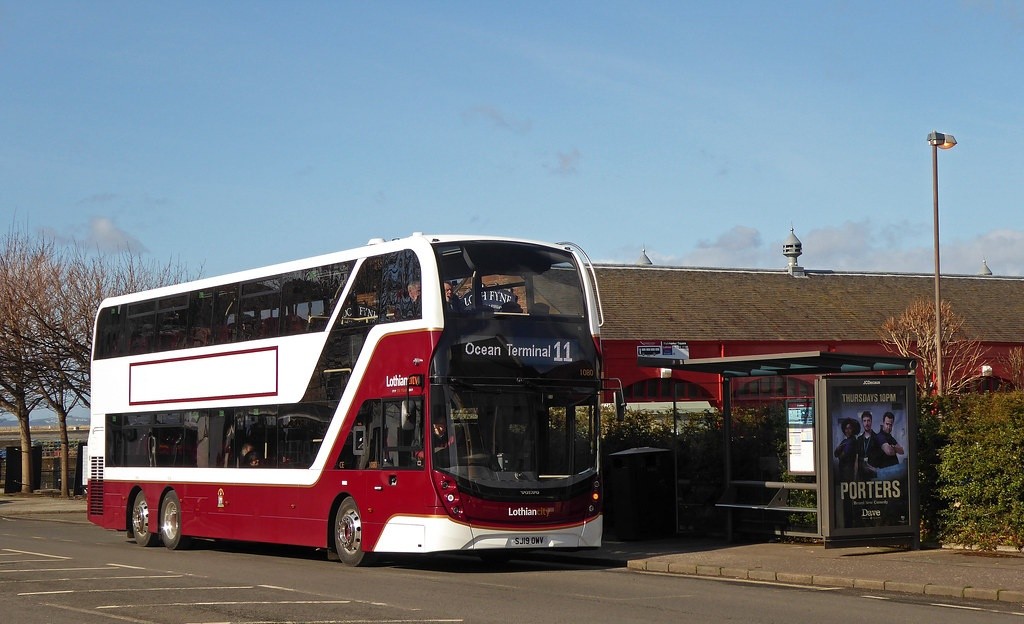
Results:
[606,446,677,542]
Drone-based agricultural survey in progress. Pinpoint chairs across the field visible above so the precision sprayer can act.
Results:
[116,313,309,357]
[499,301,550,313]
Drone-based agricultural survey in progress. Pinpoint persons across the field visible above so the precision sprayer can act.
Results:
[241,443,287,467]
[835,411,909,528]
[414,412,459,468]
[398,280,552,320]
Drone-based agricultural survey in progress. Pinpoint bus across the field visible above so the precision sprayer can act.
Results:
[84,233,628,568]
[124,424,197,466]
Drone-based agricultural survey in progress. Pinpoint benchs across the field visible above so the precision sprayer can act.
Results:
[715,479,819,512]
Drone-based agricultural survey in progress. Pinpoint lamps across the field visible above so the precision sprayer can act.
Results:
[982,366,993,376]
[661,369,672,378]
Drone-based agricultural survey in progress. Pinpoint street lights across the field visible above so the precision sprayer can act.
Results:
[927,130,958,397]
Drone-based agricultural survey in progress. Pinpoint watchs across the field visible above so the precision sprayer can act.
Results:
[891,445,894,448]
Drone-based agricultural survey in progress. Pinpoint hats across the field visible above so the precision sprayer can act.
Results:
[840,416,861,435]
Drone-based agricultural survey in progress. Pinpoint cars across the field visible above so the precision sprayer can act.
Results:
[242,427,325,468]
